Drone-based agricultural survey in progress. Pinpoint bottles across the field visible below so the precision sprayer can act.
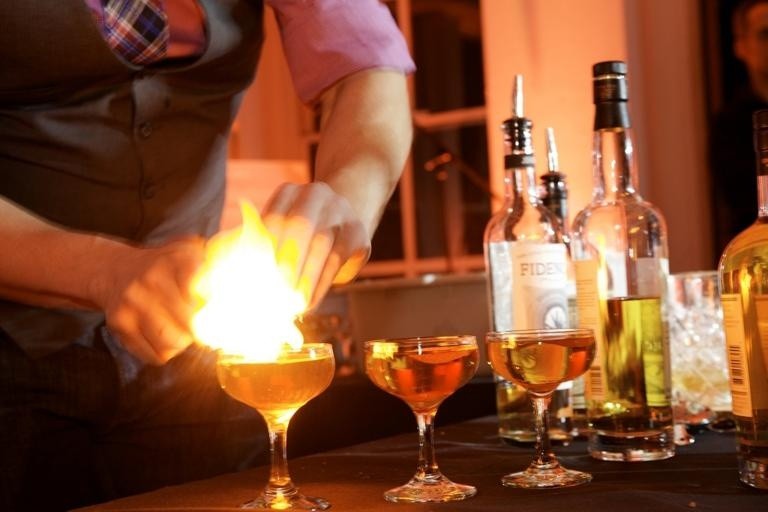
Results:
[718,7,768,494]
[568,55,678,465]
[540,167,596,444]
[488,111,577,448]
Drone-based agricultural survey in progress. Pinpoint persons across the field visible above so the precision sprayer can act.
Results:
[1,0,420,510]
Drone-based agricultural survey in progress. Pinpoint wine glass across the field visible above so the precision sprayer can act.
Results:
[215,339,337,511]
[484,322,601,489]
[364,333,482,504]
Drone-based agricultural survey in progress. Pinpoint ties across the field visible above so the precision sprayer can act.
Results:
[101,0,169,65]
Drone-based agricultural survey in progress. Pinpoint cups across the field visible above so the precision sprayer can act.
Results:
[670,266,737,434]
